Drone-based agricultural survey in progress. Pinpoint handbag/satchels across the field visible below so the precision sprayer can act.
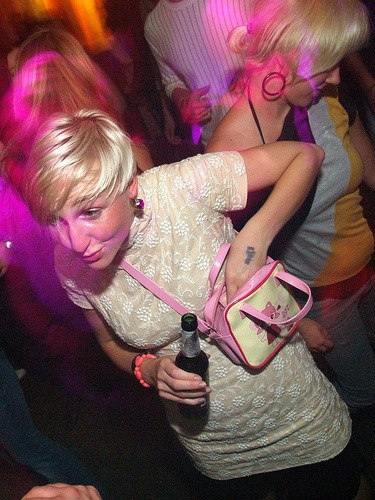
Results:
[203,242,313,369]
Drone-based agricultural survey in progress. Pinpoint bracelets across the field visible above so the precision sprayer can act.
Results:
[132,352,158,388]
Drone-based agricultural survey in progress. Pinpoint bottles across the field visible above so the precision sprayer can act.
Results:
[175,313,210,419]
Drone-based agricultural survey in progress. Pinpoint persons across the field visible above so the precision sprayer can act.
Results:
[23,106,353,500]
[0,346,107,500]
[206,0,375,415]
[0,30,152,331]
[143,0,256,147]
